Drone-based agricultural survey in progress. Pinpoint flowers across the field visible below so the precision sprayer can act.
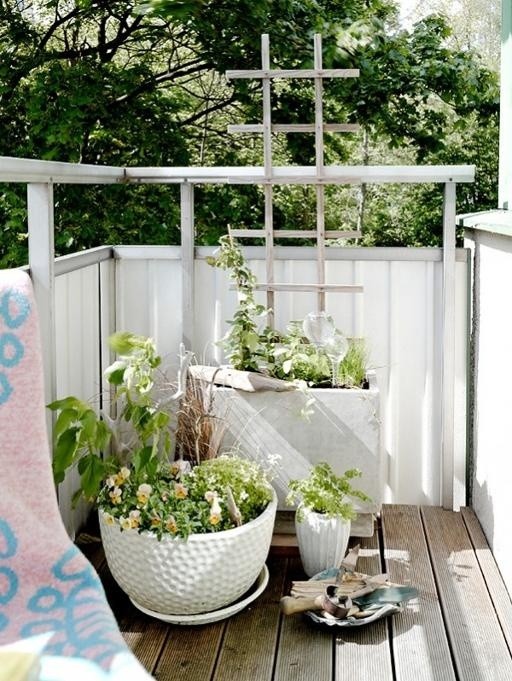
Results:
[46,333,284,540]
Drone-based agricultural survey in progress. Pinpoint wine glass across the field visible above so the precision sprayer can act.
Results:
[302,312,334,383]
[324,335,348,389]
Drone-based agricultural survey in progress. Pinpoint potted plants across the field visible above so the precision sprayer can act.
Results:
[284,463,374,583]
[195,221,387,541]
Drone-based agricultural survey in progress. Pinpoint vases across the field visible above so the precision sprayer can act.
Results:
[95,473,279,627]
